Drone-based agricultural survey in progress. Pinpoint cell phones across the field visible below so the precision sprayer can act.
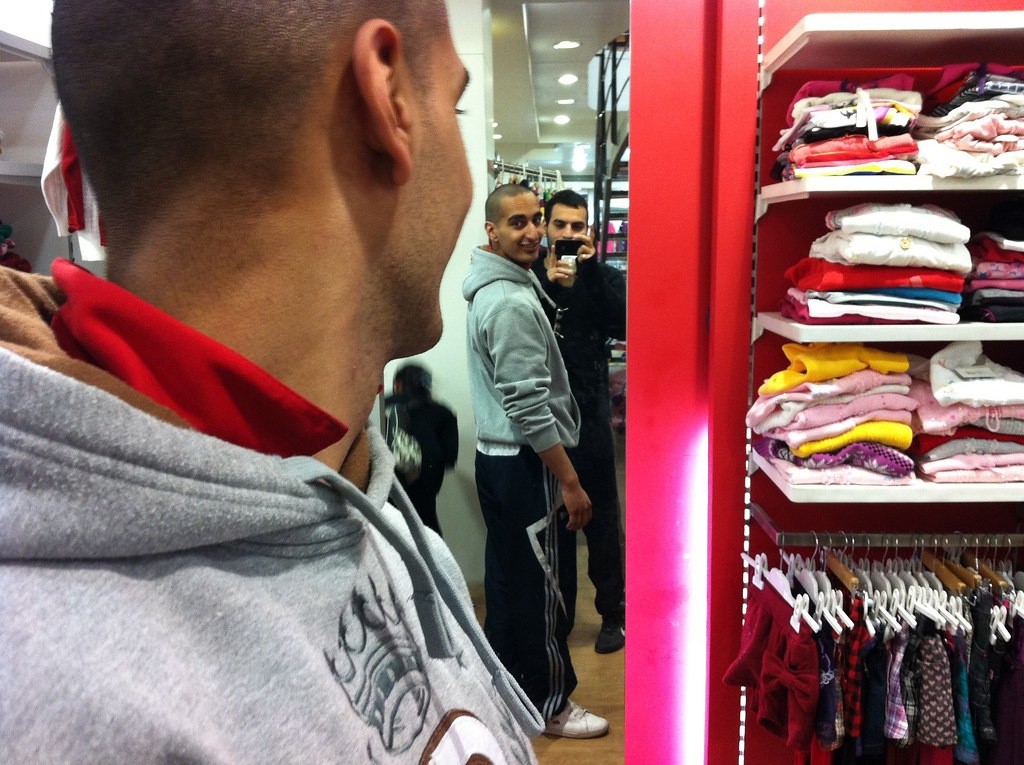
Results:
[555,239,584,256]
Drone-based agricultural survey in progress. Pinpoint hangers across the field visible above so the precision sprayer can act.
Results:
[739,529,1024,644]
[495,156,564,194]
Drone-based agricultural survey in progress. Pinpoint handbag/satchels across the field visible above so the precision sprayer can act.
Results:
[384,406,422,483]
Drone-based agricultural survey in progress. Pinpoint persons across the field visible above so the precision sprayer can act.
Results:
[460,184,609,738]
[531,190,626,654]
[384,364,458,535]
[0,0,547,765]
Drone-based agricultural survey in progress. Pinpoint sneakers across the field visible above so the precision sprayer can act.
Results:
[542,699,609,739]
[594,613,625,654]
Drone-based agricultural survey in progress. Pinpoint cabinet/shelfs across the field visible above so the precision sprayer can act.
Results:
[750,8,1024,504]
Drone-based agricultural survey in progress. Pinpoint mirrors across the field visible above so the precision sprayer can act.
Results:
[371,0,634,765]
